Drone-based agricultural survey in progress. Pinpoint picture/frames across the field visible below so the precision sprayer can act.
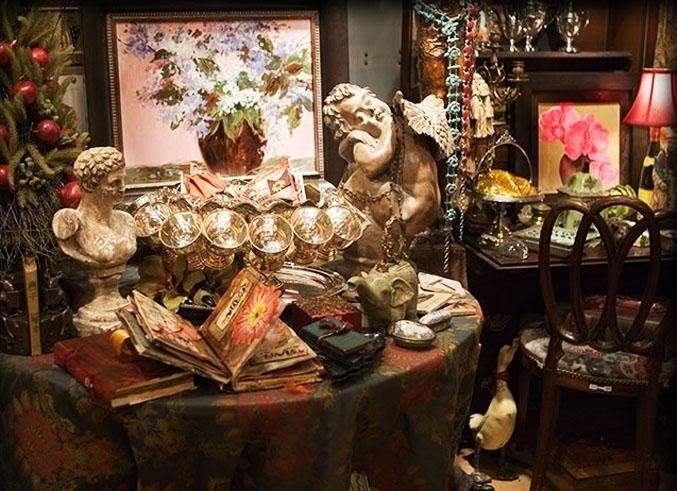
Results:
[102,9,327,187]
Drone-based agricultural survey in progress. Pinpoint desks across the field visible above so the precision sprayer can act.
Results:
[1,266,485,489]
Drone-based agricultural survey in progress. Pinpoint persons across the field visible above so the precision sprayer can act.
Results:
[320,82,444,239]
[51,145,138,268]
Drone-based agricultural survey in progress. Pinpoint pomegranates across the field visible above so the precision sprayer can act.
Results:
[0,42,82,209]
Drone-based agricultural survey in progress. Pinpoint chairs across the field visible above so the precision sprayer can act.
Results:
[523,195,672,491]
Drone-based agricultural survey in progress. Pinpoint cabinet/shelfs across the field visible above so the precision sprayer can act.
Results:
[506,66,634,206]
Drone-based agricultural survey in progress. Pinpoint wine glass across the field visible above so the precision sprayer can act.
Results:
[133,204,363,270]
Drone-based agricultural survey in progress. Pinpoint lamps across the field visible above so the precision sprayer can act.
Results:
[620,66,675,209]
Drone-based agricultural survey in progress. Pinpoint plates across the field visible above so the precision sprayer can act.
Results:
[392,320,437,349]
[510,223,601,259]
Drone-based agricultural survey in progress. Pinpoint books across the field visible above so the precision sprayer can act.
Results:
[53,327,200,408]
[114,264,327,395]
